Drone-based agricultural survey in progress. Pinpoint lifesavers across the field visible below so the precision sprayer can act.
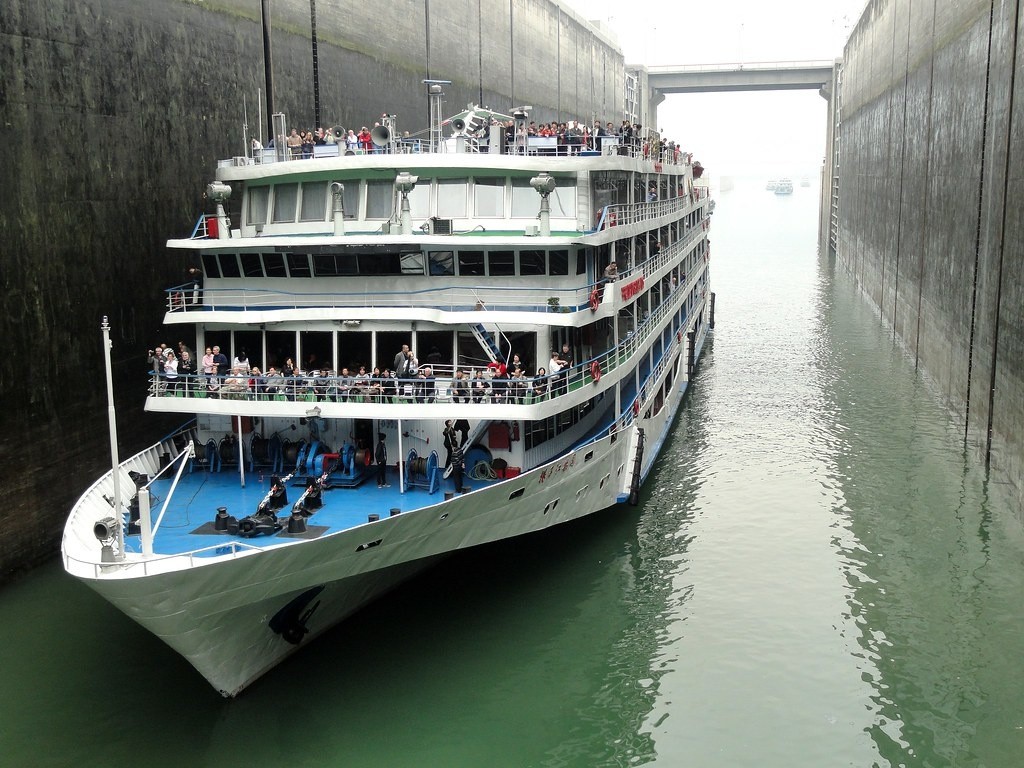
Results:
[487,363,499,377]
[591,361,600,382]
[590,290,599,311]
[597,206,617,230]
[678,331,681,343]
[644,144,648,156]
[674,152,678,162]
[635,399,638,414]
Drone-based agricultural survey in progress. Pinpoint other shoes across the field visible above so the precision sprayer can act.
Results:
[383,484,392,487]
[378,484,382,488]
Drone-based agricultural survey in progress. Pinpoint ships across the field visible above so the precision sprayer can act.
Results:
[60,79,715,700]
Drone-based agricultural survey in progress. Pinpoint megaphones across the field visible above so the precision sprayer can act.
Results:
[332,125,345,139]
[452,119,466,133]
[371,125,390,146]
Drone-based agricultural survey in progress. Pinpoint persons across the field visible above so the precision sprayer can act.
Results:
[653,242,661,255]
[647,187,656,202]
[454,419,470,447]
[450,440,466,492]
[249,113,695,167]
[597,262,619,302]
[669,277,676,292]
[375,431,392,488]
[187,264,204,304]
[147,340,574,403]
[652,188,658,201]
[680,272,685,283]
[442,420,458,469]
